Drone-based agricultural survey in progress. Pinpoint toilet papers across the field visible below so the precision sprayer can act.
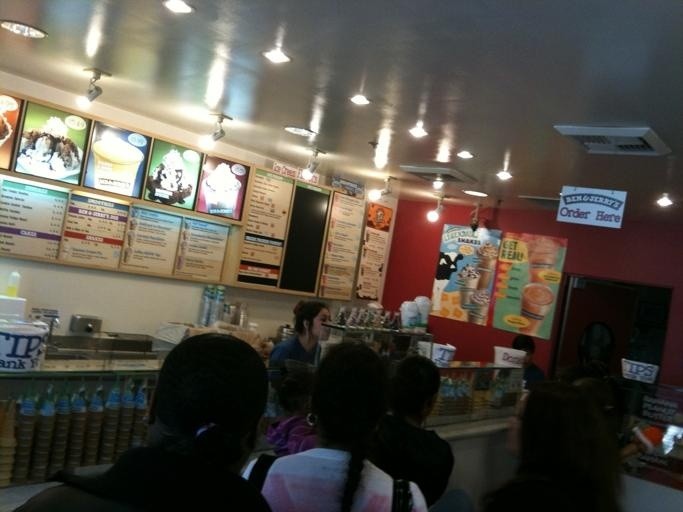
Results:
[1,296,26,322]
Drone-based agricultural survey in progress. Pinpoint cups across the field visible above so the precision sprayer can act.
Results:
[526,240,559,283]
[399,301,420,333]
[412,295,433,333]
[519,283,554,336]
[468,295,491,327]
[249,322,258,331]
[91,141,144,198]
[457,277,479,308]
[202,178,241,219]
[475,250,498,290]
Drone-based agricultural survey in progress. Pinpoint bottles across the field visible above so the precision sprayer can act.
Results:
[198,285,250,329]
[6,269,20,298]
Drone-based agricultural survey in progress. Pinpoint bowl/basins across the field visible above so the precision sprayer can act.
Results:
[493,345,528,368]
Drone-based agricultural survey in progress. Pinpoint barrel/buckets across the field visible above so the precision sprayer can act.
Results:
[0,324,49,373]
[494,344,530,366]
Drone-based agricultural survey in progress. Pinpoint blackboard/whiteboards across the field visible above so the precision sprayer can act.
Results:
[276,179,334,298]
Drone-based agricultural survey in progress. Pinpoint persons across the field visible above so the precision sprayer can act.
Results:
[497,334,545,389]
[241,342,454,510]
[480,362,633,511]
[264,298,332,402]
[12,331,271,511]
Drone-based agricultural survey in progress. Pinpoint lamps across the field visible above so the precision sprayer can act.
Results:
[83,67,113,102]
[380,175,398,196]
[470,203,482,231]
[302,148,326,180]
[209,111,233,142]
[427,195,446,222]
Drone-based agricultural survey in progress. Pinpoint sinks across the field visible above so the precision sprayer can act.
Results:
[45,352,93,361]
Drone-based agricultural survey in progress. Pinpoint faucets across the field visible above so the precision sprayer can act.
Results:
[43,317,61,359]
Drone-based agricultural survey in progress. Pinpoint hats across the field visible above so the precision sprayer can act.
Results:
[151,331,270,434]
